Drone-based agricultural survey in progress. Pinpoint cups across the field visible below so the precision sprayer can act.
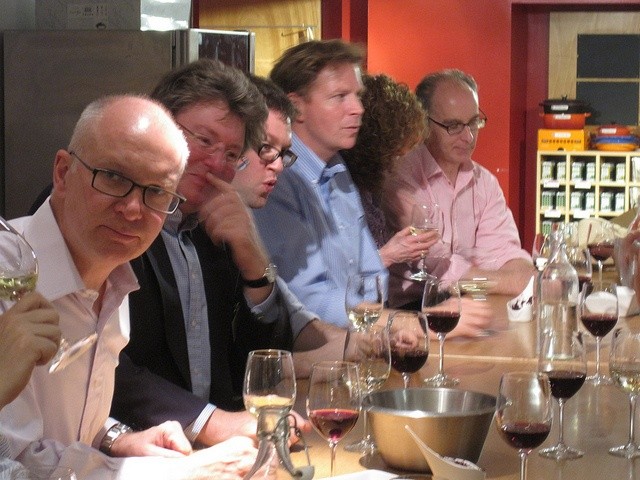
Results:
[11,466,78,480]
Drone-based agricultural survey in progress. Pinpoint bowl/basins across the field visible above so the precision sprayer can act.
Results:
[362,387,498,472]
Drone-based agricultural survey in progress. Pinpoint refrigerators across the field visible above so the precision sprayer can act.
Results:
[1,30,258,215]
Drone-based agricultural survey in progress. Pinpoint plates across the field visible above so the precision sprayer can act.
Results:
[592,135,638,144]
[593,144,637,151]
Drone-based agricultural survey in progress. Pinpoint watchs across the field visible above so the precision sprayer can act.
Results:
[99,422,133,456]
[242,262,279,289]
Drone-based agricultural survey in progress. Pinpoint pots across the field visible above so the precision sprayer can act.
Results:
[538,113,593,129]
[538,94,592,113]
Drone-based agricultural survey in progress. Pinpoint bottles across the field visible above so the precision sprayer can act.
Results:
[534,229,579,360]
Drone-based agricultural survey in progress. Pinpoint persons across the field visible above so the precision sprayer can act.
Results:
[27,57,314,461]
[337,71,443,271]
[229,71,420,379]
[616,196,640,309]
[248,38,496,343]
[0,291,63,413]
[1,91,278,480]
[374,68,535,313]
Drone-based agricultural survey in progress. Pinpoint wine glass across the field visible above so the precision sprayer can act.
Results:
[243,349,298,480]
[578,279,618,386]
[558,221,580,264]
[496,372,553,480]
[421,277,462,388]
[388,309,430,387]
[606,329,640,459]
[305,361,361,479]
[459,246,500,340]
[409,204,442,284]
[536,330,589,460]
[345,275,384,381]
[340,325,391,453]
[535,229,560,272]
[586,221,617,279]
[0,216,98,375]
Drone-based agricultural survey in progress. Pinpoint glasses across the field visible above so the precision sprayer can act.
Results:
[178,118,249,174]
[65,145,186,214]
[256,143,300,170]
[425,105,490,137]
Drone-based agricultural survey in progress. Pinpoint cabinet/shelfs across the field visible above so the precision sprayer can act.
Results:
[537,150,640,242]
